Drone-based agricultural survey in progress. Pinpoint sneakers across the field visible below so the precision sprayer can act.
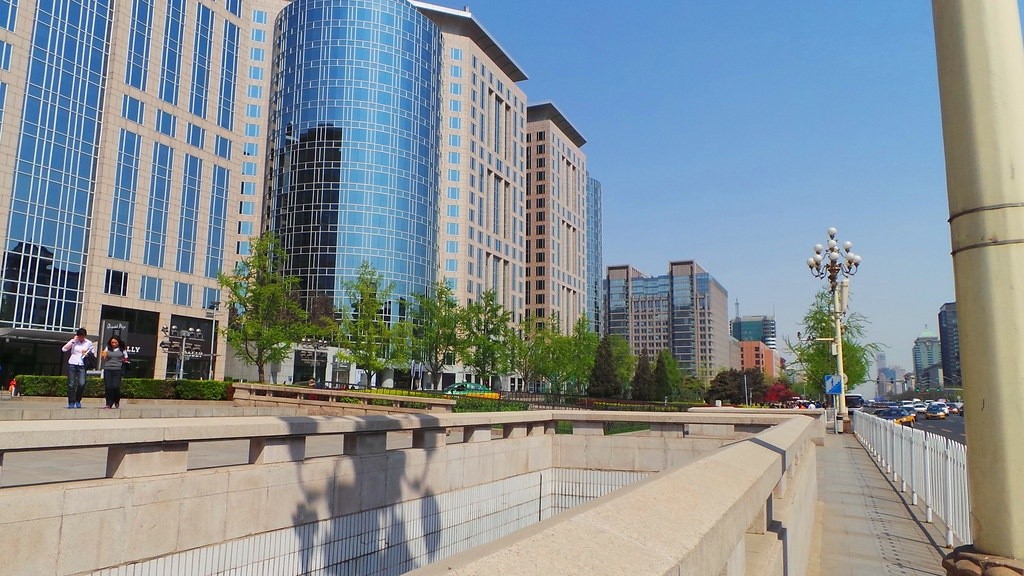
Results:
[68,402,81,409]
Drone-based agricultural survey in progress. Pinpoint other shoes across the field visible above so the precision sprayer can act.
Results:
[105,403,119,409]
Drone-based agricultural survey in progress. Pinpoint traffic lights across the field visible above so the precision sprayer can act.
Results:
[914,387,921,393]
[925,386,930,393]
[936,387,941,393]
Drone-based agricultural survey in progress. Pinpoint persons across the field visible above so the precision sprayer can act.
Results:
[101,335,129,409]
[308,376,327,389]
[62,327,94,409]
[762,399,824,409]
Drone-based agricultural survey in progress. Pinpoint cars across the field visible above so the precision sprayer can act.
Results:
[438,379,501,401]
[864,393,964,418]
[869,409,886,418]
[902,406,918,420]
[925,403,946,421]
[879,406,915,428]
[291,380,329,389]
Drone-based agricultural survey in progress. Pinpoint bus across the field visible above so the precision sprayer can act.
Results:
[844,393,865,415]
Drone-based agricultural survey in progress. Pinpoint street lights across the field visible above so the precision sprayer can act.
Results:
[805,227,869,434]
[211,298,222,380]
[300,336,333,381]
[157,323,205,380]
